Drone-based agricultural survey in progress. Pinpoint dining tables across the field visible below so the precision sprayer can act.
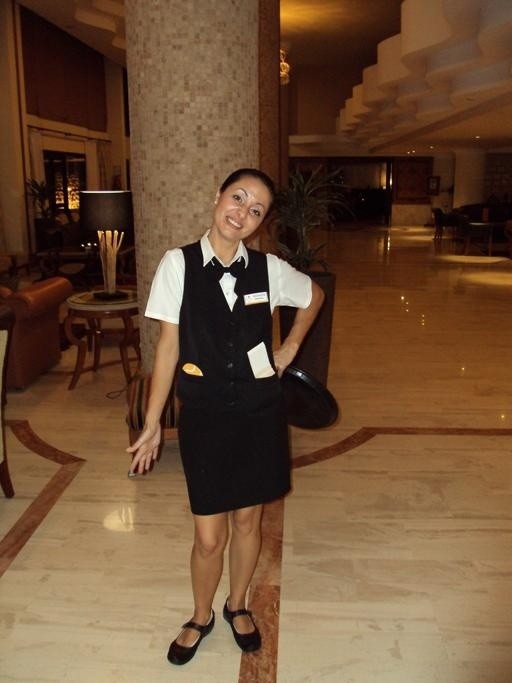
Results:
[269,163,356,389]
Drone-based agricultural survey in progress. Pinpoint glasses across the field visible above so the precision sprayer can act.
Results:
[280,46,291,87]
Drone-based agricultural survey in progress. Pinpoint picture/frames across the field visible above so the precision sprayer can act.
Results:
[36,245,134,285]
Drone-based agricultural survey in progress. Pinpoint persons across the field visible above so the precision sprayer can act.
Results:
[126,169,326,666]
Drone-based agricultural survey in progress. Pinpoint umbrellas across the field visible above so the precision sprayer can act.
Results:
[222,598,261,651]
[167,607,215,665]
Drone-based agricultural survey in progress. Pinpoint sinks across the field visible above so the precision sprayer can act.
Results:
[1,277,73,389]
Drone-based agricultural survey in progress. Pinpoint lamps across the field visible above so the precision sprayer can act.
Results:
[430,202,512,255]
[1,308,16,498]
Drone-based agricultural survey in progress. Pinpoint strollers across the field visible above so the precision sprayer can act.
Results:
[206,257,245,287]
